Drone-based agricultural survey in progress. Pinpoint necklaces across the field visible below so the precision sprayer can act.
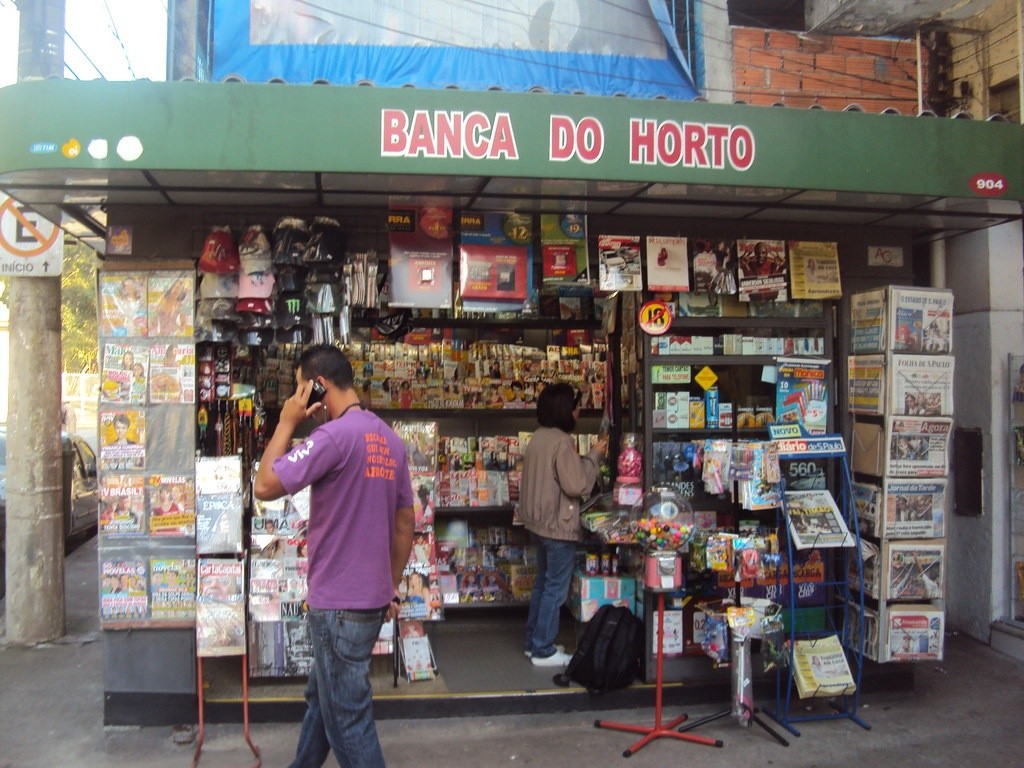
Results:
[337,402,362,420]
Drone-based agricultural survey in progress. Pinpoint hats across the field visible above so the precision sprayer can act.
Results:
[197,213,341,348]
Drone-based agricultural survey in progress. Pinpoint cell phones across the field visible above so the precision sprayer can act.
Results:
[307,380,326,409]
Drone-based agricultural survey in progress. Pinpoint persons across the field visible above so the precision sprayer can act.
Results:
[253,345,413,768]
[110,274,952,658]
[518,382,610,666]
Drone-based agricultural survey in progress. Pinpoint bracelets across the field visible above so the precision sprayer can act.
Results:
[394,597,401,604]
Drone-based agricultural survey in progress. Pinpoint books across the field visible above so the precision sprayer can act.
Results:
[100,231,859,701]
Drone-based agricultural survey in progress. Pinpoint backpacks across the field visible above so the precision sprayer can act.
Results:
[561,604,642,705]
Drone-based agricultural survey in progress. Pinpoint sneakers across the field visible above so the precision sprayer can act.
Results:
[524,643,565,657]
[530,648,573,667]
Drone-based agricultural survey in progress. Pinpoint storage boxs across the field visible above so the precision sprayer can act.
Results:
[800,338,811,354]
[773,337,784,355]
[701,336,713,355]
[742,337,753,355]
[719,334,733,355]
[793,338,800,354]
[737,408,755,428]
[783,338,793,354]
[653,386,732,429]
[680,337,691,354]
[763,338,773,354]
[649,337,659,355]
[732,334,742,354]
[713,337,724,356]
[811,338,825,355]
[753,338,764,354]
[691,336,702,354]
[659,336,670,355]
[756,407,772,429]
[669,335,680,354]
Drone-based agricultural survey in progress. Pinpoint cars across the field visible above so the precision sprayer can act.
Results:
[0,433,97,553]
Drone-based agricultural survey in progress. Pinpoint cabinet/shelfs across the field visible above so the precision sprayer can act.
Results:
[264,404,605,609]
[845,284,955,664]
[611,315,836,681]
[771,437,871,735]
